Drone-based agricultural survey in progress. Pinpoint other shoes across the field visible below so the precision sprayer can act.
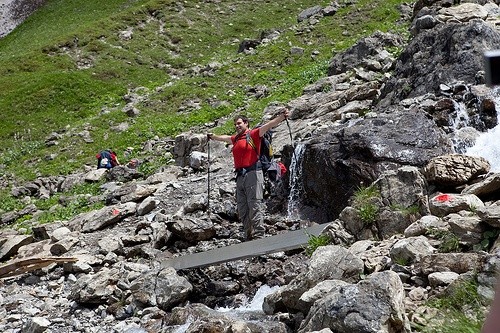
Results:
[245,235,264,242]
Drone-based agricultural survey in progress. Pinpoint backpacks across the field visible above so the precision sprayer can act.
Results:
[253,124,273,173]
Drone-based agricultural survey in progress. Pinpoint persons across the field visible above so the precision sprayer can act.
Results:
[206,108,291,242]
